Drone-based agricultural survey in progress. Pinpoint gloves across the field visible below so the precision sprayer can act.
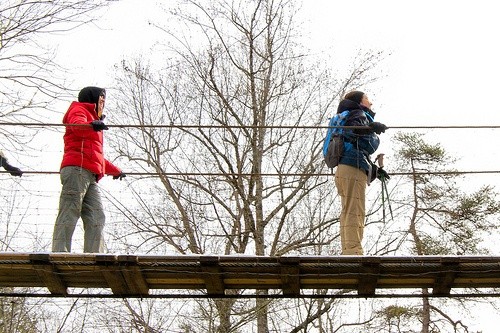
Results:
[365,121,387,135]
[9,167,22,176]
[113,172,126,180]
[90,121,108,131]
[372,165,390,182]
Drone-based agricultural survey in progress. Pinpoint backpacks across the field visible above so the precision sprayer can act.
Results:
[322,109,374,168]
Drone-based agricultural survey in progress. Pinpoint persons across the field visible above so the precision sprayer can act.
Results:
[51,87,126,253]
[335,91,391,255]
[0,151,22,177]
